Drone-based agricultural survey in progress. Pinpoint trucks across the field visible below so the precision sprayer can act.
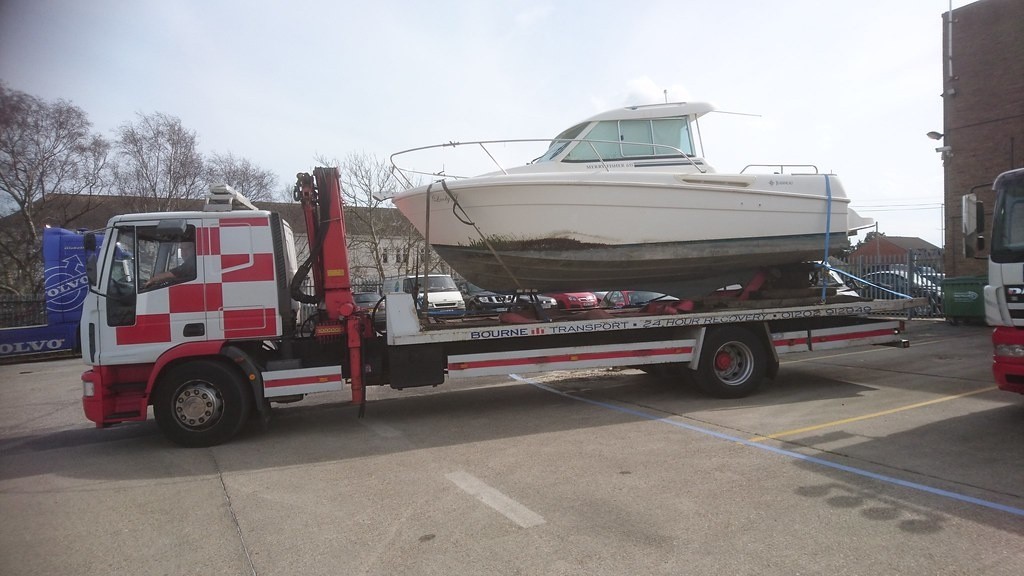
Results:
[79,166,935,449]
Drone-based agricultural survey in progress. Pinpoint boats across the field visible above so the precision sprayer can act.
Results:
[365,93,881,296]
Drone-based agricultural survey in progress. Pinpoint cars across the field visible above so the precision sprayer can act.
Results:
[807,256,945,313]
[381,274,466,321]
[468,285,679,315]
[353,291,387,325]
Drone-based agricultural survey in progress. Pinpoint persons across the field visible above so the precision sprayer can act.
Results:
[144,242,196,286]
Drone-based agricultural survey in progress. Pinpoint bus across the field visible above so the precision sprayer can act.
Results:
[962,168,1024,400]
